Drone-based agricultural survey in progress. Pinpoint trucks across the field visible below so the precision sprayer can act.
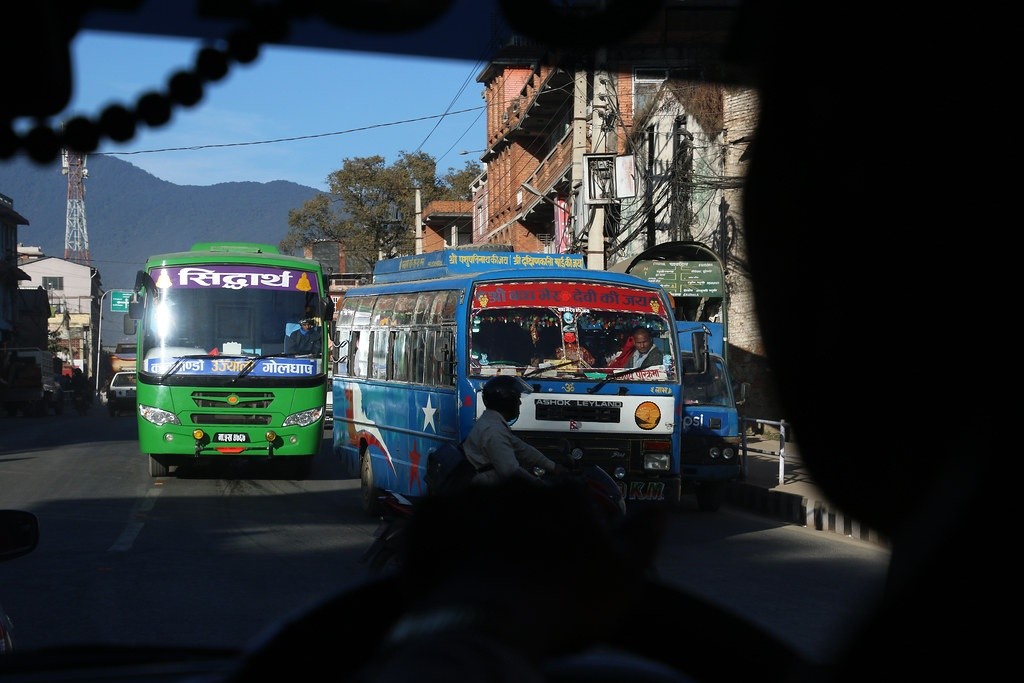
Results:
[0,347,65,416]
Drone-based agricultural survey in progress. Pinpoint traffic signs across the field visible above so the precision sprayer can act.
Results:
[110,291,134,312]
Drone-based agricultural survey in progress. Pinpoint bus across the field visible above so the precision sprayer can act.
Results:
[128,242,334,477]
[324,243,740,530]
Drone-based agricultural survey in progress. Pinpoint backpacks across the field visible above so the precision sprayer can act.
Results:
[424,434,475,511]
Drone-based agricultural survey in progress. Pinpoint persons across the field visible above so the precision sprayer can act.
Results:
[71,368,94,406]
[463,376,575,499]
[285,317,323,360]
[622,326,666,375]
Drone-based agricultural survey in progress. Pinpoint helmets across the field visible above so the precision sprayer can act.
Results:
[482,375,534,421]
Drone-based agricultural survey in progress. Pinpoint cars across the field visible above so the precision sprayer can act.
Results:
[110,371,138,417]
[112,343,136,370]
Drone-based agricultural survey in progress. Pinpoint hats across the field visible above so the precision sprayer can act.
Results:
[563,324,576,334]
